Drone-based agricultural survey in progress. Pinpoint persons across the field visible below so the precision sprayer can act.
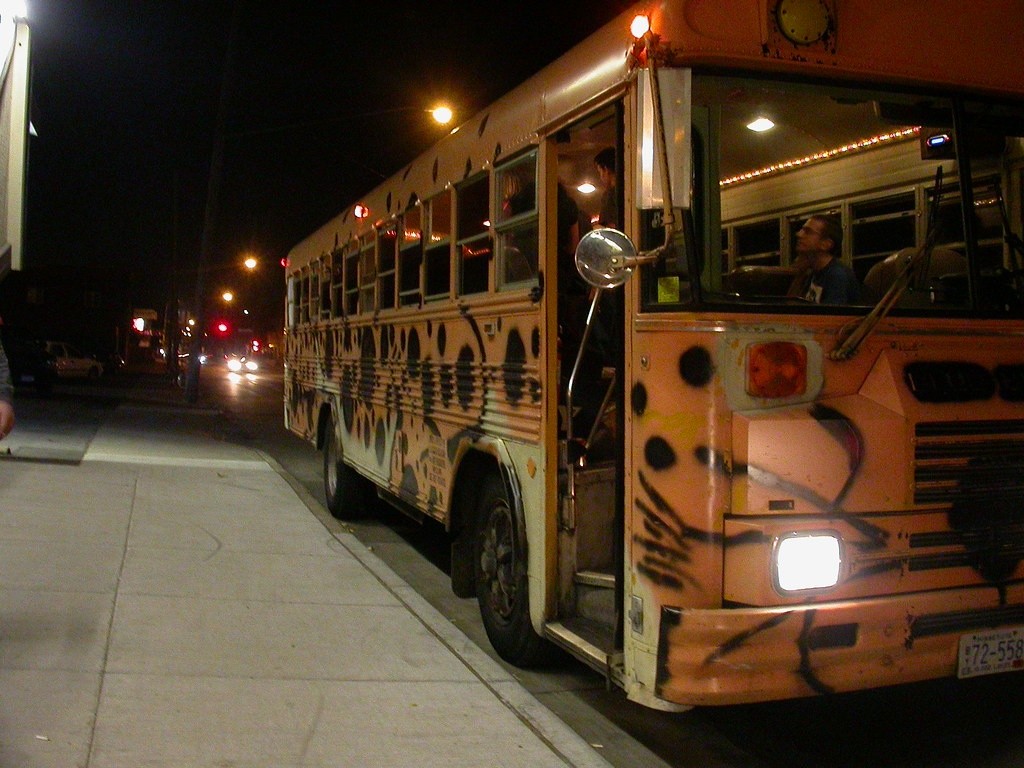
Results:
[510,147,625,437]
[785,214,864,305]
[0,340,17,441]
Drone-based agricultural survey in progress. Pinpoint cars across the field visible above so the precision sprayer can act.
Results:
[196,351,272,371]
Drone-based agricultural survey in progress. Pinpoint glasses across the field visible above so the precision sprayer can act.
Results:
[800,225,829,240]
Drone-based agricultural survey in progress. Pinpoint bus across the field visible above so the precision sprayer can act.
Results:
[271,1,1024,714]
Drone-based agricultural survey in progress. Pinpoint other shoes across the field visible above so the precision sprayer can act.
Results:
[603,400,617,415]
